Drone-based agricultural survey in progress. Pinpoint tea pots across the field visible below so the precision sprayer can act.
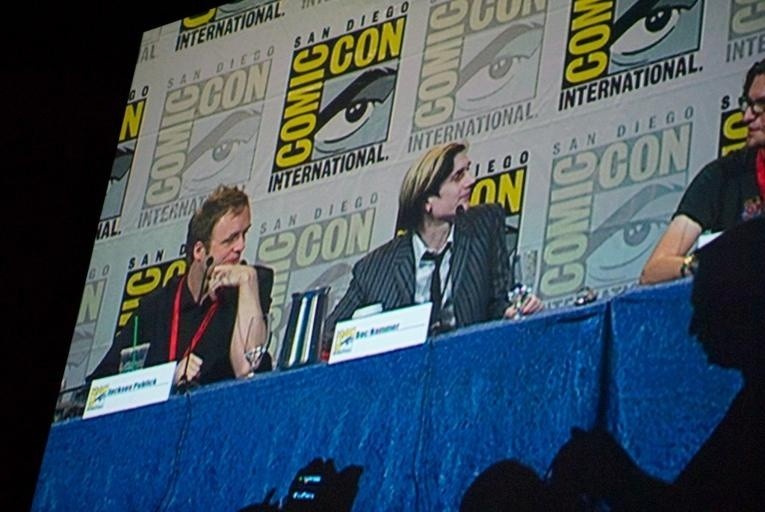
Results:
[277,287,334,371]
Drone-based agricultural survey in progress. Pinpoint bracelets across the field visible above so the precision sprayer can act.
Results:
[679,255,695,276]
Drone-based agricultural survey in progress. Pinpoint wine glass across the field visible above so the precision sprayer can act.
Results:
[242,315,271,382]
[506,251,537,322]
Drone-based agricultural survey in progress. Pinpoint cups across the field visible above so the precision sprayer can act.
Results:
[118,344,149,371]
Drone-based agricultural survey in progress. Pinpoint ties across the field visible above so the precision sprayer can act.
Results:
[420,239,454,326]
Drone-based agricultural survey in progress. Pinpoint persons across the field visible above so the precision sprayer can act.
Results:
[635,55,765,291]
[320,137,545,358]
[60,181,275,408]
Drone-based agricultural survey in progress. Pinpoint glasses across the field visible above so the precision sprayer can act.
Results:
[737,94,765,116]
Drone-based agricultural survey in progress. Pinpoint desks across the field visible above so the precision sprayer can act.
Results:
[33,274,745,507]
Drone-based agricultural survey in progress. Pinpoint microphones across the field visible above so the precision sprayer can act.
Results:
[181,258,214,383]
[435,206,464,320]
